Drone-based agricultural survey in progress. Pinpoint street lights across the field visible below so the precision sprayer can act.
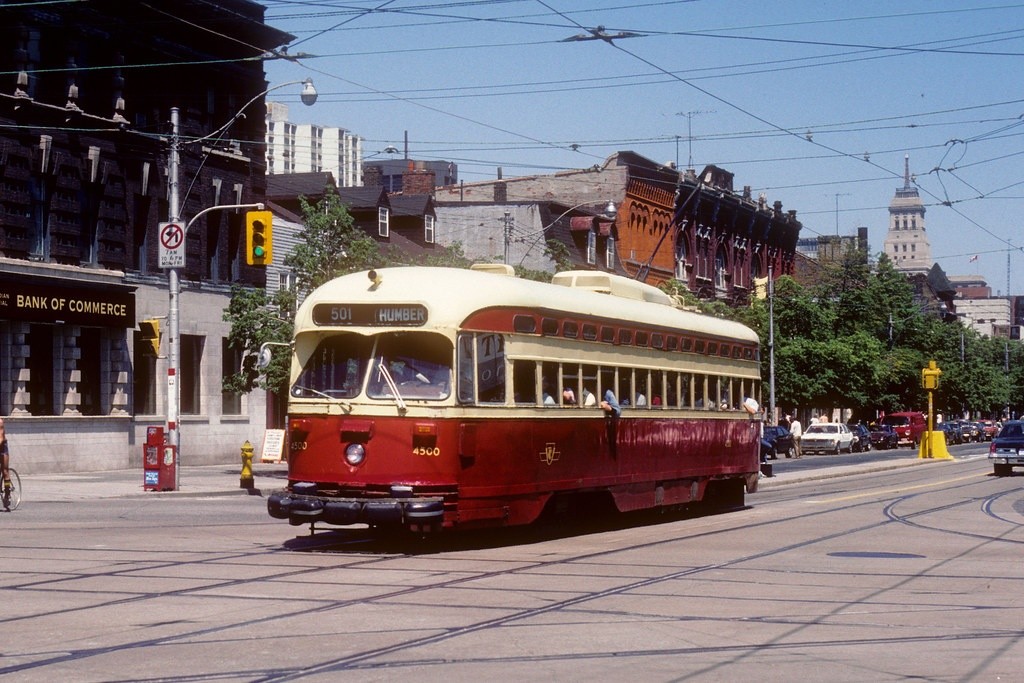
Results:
[502,199,616,278]
[888,302,948,375]
[169,77,319,490]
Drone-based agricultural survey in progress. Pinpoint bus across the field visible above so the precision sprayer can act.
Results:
[257,182,763,536]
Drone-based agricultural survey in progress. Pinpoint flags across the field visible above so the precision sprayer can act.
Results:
[970,255,977,262]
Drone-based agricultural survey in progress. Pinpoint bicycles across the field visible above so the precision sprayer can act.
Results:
[0,455,22,511]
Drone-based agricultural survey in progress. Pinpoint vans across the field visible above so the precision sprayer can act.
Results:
[879,412,927,450]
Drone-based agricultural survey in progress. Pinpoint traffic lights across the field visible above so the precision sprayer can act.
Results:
[246,211,273,265]
[1008,325,1024,341]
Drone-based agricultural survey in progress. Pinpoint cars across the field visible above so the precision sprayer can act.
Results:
[801,423,899,455]
[988,420,1024,476]
[936,419,1002,446]
[763,426,794,459]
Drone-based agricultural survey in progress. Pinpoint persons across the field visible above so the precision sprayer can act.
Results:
[761,405,765,414]
[0,418,10,506]
[583,379,596,405]
[976,421,984,443]
[600,389,621,418]
[563,386,576,404]
[947,427,962,446]
[651,391,662,405]
[720,397,727,409]
[736,388,759,414]
[696,389,714,407]
[778,411,802,458]
[811,411,828,432]
[636,392,646,405]
[542,376,555,404]
[667,381,676,405]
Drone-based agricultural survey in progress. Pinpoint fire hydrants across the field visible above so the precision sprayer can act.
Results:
[240,440,254,488]
